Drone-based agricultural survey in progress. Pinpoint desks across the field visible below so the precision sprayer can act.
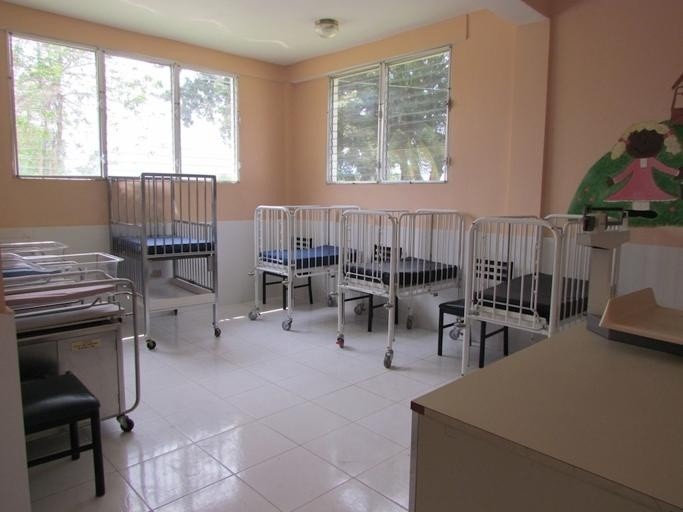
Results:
[408,327,683,508]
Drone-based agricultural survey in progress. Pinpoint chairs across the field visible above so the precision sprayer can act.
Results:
[20,369,105,499]
[437,257,515,369]
[261,232,314,310]
[336,243,403,332]
